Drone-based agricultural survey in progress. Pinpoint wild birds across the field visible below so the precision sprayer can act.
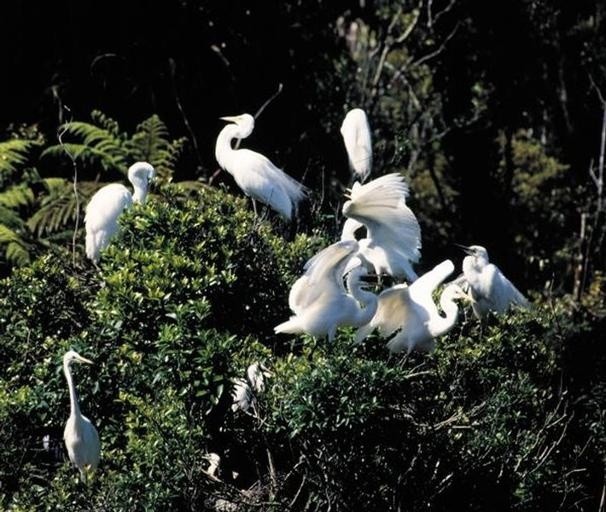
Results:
[214,111,310,226]
[83,160,155,268]
[340,108,374,185]
[272,173,531,368]
[60,349,100,485]
